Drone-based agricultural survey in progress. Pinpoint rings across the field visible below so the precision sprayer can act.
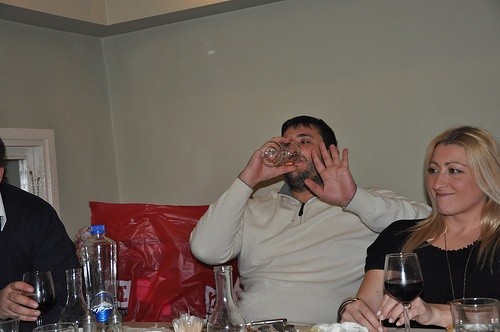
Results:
[407,305,413,310]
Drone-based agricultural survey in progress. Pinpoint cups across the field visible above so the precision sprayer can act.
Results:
[448,297,500,332]
[31,321,79,332]
[0,314,20,332]
[259,141,300,167]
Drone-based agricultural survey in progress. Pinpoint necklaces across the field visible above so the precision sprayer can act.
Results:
[443,232,482,303]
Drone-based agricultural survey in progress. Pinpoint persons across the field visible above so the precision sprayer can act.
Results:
[188,116,433,325]
[0,138,88,332]
[336,124,499,332]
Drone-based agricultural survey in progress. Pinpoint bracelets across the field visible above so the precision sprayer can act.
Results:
[338,296,358,322]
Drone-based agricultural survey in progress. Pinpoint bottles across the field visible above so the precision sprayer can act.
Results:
[206,264,248,332]
[80,224,117,332]
[57,267,86,322]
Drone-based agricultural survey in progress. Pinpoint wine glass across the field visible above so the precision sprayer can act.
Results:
[382,252,425,332]
[21,270,57,328]
